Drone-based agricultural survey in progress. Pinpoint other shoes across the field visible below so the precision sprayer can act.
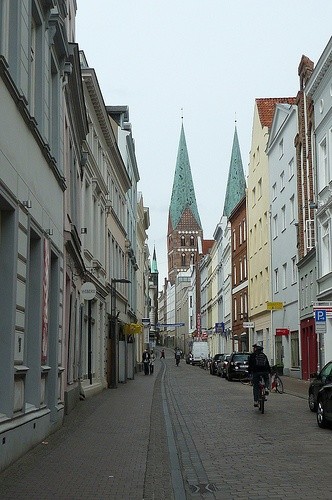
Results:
[265,391,269,395]
[254,403,259,407]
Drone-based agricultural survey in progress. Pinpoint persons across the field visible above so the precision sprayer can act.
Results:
[160,350,165,359]
[248,343,271,407]
[142,349,150,375]
[174,348,182,366]
[150,350,156,372]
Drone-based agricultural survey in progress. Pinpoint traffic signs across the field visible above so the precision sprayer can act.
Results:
[315,310,327,334]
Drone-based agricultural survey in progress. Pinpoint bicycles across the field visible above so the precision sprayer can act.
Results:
[239,370,252,385]
[246,370,271,414]
[267,364,284,394]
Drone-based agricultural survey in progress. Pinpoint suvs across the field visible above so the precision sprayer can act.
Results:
[224,351,252,382]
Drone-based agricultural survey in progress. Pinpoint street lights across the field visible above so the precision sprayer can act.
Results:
[111,278,131,388]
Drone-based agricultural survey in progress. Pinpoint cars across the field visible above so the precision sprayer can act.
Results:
[308,360,332,412]
[200,353,231,378]
[316,384,332,429]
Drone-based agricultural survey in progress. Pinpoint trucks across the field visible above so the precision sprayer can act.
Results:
[185,341,210,366]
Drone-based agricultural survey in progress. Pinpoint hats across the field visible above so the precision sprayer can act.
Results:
[252,343,263,351]
